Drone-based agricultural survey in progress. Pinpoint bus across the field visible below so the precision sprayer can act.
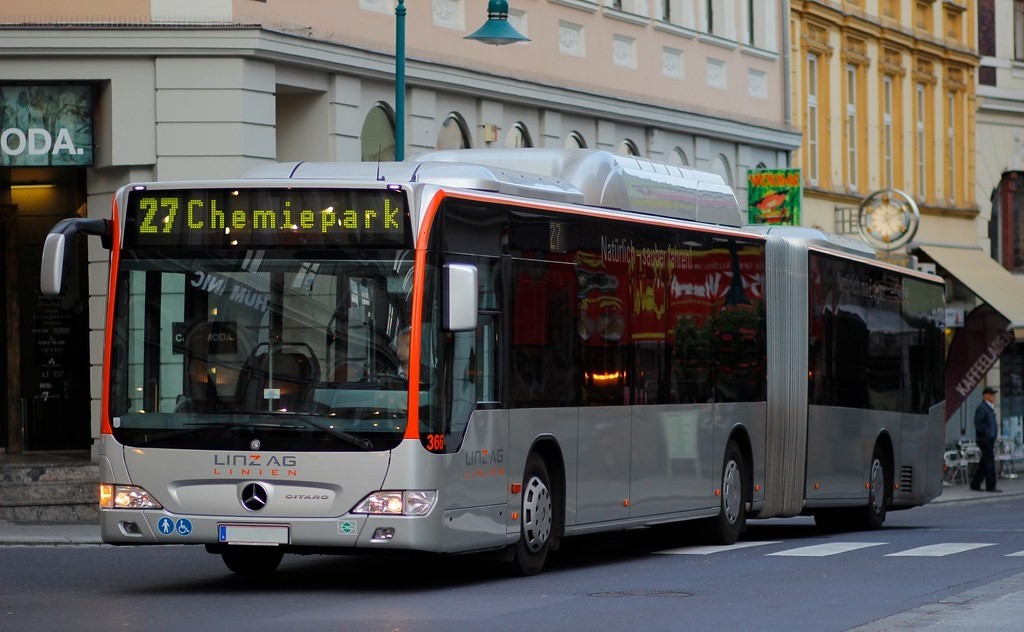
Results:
[40,148,948,577]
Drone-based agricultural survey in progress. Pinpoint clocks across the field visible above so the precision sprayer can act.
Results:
[856,189,921,252]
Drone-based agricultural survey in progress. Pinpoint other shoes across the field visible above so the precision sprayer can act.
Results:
[970,486,984,491]
[986,489,1002,492]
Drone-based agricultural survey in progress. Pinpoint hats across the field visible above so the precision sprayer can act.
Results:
[982,387,998,395]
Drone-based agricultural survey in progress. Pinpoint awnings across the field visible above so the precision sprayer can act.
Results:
[914,240,1024,340]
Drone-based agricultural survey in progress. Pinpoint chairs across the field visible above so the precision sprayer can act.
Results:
[943,435,1024,488]
[237,340,321,408]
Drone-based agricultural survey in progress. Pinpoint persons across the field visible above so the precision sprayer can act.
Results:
[384,330,431,393]
[969,385,1002,493]
